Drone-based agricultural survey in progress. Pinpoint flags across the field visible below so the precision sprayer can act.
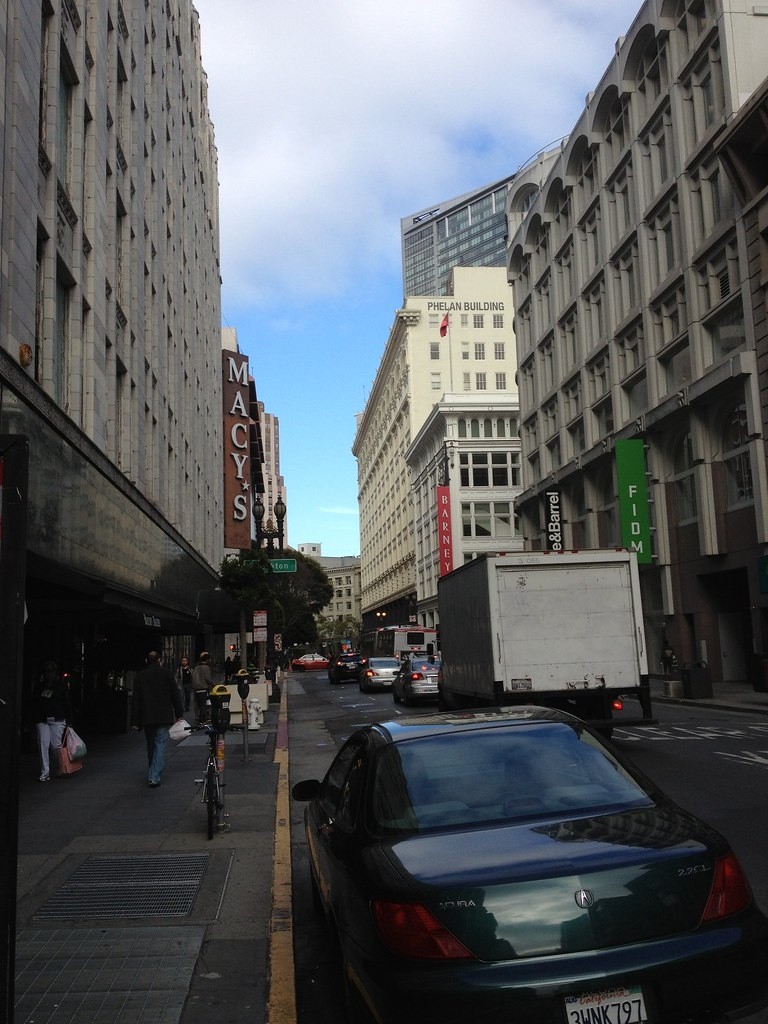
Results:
[440,314,448,337]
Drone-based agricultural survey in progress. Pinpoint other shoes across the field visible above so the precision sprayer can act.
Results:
[40,774,50,781]
[148,779,160,786]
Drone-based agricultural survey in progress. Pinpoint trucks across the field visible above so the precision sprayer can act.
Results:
[436,548,661,741]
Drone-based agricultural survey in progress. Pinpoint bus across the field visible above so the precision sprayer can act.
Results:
[367,624,438,658]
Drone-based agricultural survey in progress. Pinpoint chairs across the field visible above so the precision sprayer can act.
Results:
[501,756,546,805]
[401,752,447,811]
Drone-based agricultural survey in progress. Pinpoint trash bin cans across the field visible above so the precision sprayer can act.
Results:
[682,661,713,698]
[101,689,130,734]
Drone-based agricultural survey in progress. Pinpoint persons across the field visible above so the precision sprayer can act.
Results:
[176,657,193,712]
[130,651,183,787]
[191,654,212,722]
[32,661,72,782]
[660,649,678,680]
[223,654,241,681]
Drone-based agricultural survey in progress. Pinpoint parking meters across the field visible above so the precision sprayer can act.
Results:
[208,684,232,834]
[235,668,253,764]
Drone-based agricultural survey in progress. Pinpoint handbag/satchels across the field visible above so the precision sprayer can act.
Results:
[66,726,87,761]
[169,720,191,740]
[59,726,83,774]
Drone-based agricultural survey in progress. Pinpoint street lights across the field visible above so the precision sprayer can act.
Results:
[251,495,287,707]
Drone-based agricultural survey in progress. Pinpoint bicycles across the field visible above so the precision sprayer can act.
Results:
[183,717,227,840]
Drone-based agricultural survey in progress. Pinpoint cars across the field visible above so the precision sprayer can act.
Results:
[291,653,329,672]
[292,701,768,1024]
[327,652,364,685]
[357,656,405,694]
[390,658,441,708]
